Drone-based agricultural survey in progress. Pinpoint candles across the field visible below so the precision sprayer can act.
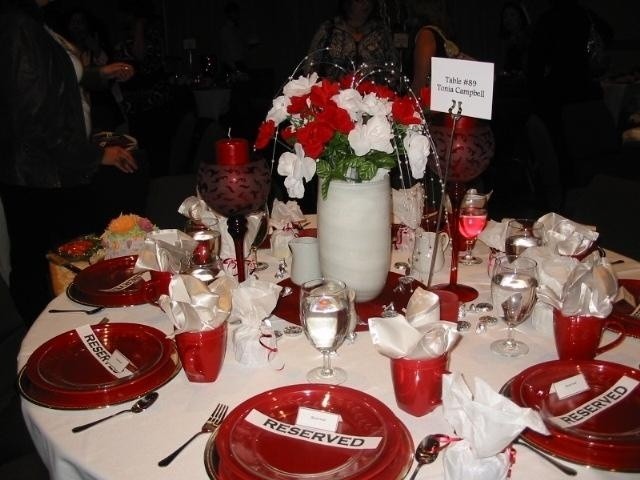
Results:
[215,127,249,166]
[446,108,477,135]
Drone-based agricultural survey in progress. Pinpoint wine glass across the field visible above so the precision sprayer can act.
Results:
[246,205,269,272]
[504,219,548,261]
[458,193,491,267]
[301,278,351,386]
[489,256,540,358]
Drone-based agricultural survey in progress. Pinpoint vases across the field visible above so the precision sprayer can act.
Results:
[314,165,395,304]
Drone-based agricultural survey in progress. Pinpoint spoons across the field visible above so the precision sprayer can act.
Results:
[68,392,163,432]
[493,429,577,477]
[407,434,439,480]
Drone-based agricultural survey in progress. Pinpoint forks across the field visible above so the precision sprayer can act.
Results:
[159,401,227,468]
[48,306,107,316]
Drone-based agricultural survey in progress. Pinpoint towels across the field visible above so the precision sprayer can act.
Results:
[181,194,266,261]
[528,248,624,324]
[132,230,201,273]
[526,208,600,254]
[368,286,462,364]
[156,265,233,336]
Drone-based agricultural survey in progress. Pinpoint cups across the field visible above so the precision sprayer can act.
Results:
[286,236,323,287]
[430,290,458,323]
[412,230,449,274]
[183,216,221,265]
[389,353,451,416]
[172,321,228,383]
[551,306,629,359]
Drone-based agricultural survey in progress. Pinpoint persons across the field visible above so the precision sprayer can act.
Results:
[0,1,640,332]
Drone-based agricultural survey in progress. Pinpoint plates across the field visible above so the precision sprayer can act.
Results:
[216,384,404,480]
[511,358,639,448]
[589,275,640,337]
[26,325,170,395]
[71,254,175,306]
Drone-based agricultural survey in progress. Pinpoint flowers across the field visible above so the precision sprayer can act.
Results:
[258,33,447,203]
